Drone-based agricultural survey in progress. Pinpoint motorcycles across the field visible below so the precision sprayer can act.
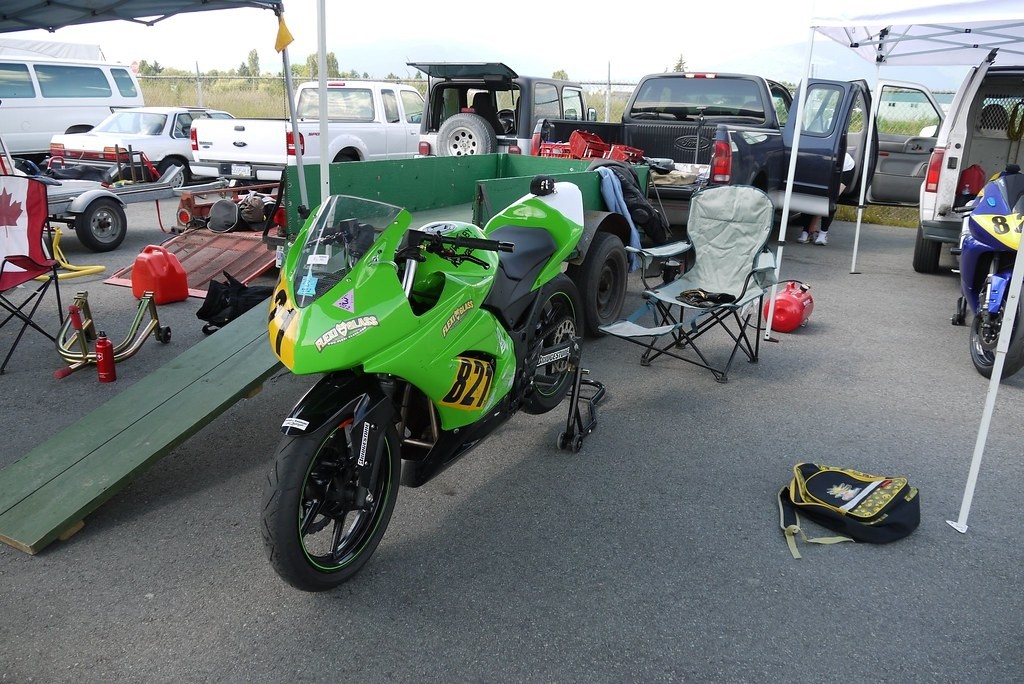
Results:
[258,181,586,594]
[950,164,1024,379]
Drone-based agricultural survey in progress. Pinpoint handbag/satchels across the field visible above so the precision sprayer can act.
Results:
[196,270,275,334]
[206,190,284,233]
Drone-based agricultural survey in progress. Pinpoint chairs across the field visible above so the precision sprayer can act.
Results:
[471,92,506,135]
[597,184,779,383]
[0,174,66,375]
[980,105,1009,131]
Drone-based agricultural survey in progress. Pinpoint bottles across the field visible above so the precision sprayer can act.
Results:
[132,245,188,305]
[95,330,117,383]
[959,184,970,205]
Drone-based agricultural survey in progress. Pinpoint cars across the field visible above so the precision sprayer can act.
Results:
[49,106,237,189]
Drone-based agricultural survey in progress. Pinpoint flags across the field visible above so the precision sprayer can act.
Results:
[275,15,294,53]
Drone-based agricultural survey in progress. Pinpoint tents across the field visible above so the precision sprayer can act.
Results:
[763,0,1024,533]
[0,0,310,209]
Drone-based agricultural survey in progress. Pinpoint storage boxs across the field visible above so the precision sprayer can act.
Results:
[540,143,579,159]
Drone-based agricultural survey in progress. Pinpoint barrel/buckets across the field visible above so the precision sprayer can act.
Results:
[957,164,985,195]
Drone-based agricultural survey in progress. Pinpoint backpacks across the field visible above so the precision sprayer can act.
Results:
[777,462,920,559]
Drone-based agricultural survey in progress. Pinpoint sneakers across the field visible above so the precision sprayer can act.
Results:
[797,231,814,243]
[814,230,828,245]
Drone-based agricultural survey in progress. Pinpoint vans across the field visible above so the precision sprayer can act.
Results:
[865,47,1024,274]
[0,58,145,155]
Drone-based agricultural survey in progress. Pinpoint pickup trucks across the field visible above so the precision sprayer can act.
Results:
[188,80,425,181]
[530,71,880,227]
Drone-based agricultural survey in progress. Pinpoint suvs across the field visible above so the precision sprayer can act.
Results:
[405,61,597,159]
